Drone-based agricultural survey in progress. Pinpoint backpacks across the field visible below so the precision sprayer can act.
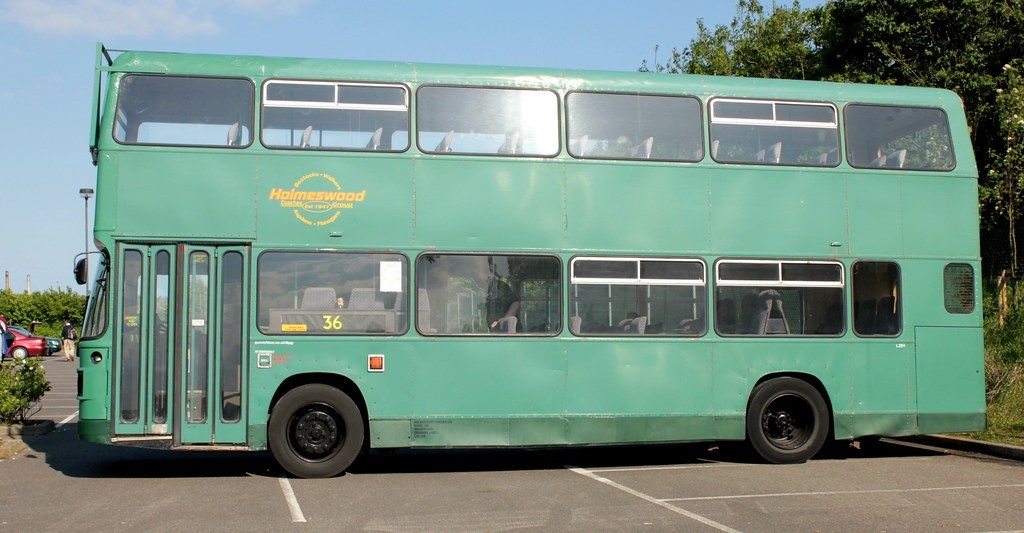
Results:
[67,326,77,340]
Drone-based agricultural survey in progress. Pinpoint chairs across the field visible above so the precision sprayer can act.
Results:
[815,148,839,164]
[368,127,383,149]
[718,290,792,334]
[571,135,588,157]
[752,142,782,163]
[348,288,386,309]
[298,286,335,310]
[571,315,665,335]
[435,131,455,154]
[692,140,719,159]
[674,317,703,335]
[228,123,241,146]
[815,297,899,336]
[497,316,517,334]
[392,288,431,333]
[495,132,520,154]
[871,149,906,167]
[300,126,313,148]
[626,137,652,159]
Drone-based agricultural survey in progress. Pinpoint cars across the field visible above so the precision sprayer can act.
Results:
[5,324,62,356]
[7,327,49,361]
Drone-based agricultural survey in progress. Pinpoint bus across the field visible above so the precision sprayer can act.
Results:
[72,42,988,478]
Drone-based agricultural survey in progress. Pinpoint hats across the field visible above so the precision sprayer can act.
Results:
[65,321,70,326]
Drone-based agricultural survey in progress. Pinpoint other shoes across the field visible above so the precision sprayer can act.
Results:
[71,357,74,362]
[67,359,70,361]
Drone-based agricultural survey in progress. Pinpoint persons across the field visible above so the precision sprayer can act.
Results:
[626,311,640,319]
[580,312,592,332]
[0,313,10,371]
[61,320,76,362]
[466,262,524,333]
